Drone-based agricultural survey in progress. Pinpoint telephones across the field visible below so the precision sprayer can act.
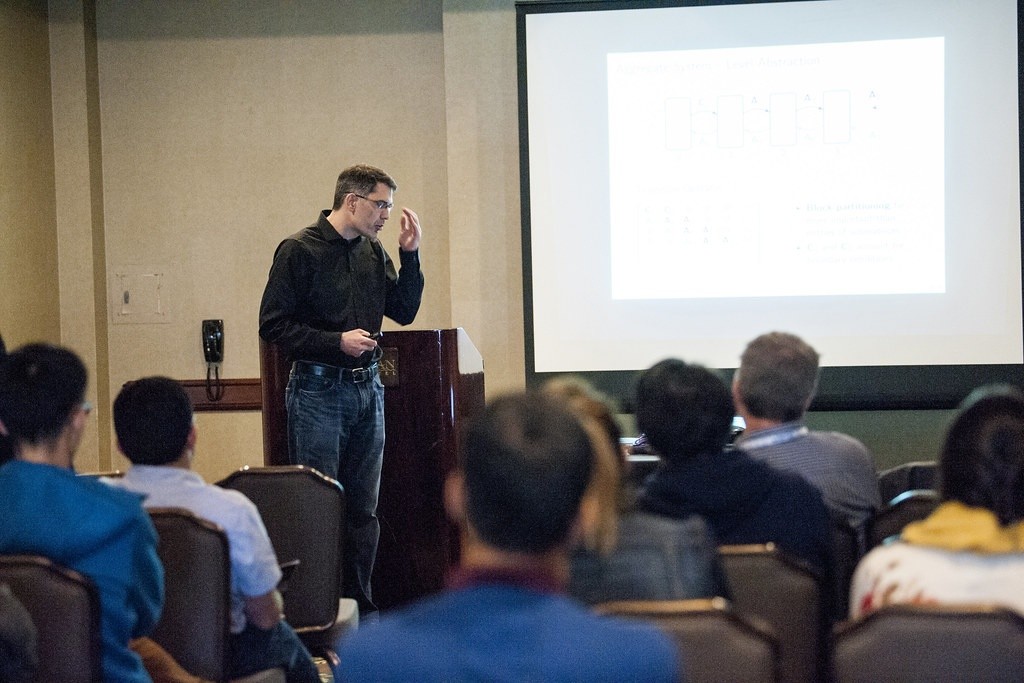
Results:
[201,319,224,363]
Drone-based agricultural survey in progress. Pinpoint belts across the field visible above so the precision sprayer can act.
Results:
[291,361,379,383]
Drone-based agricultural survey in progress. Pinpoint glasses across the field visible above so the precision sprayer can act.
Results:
[81,402,93,415]
[343,191,394,211]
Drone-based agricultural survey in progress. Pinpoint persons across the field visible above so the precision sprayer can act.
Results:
[0,333,1023,683]
[258,164,424,617]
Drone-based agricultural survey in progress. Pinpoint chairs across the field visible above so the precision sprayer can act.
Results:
[0,466,344,683]
[593,460,1024,683]
